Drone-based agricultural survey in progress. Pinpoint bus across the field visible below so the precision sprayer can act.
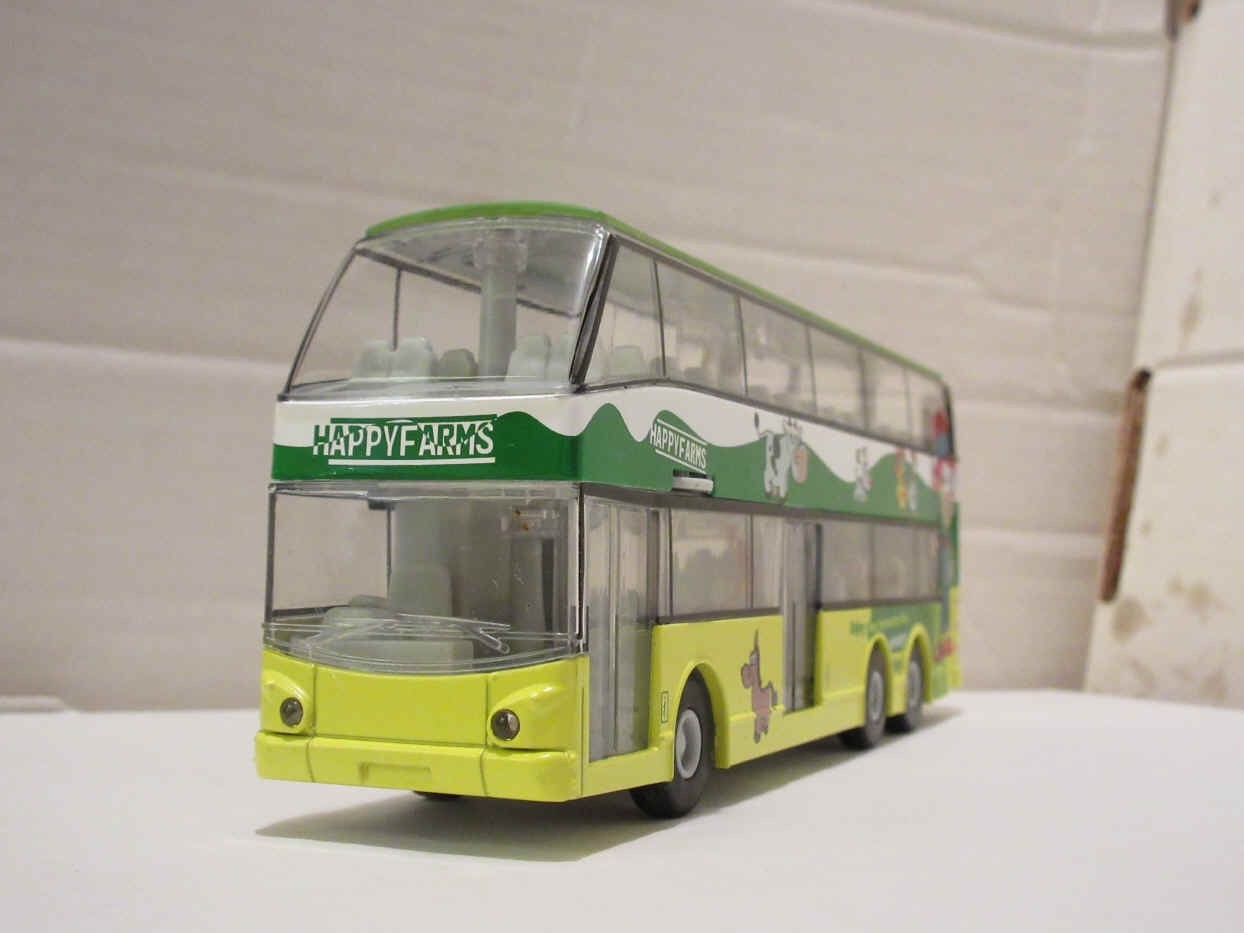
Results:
[252,200,966,819]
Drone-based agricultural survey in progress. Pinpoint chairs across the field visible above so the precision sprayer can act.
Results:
[388,563,452,621]
[877,557,906,598]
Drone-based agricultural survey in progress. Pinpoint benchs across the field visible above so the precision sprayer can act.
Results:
[355,332,910,446]
